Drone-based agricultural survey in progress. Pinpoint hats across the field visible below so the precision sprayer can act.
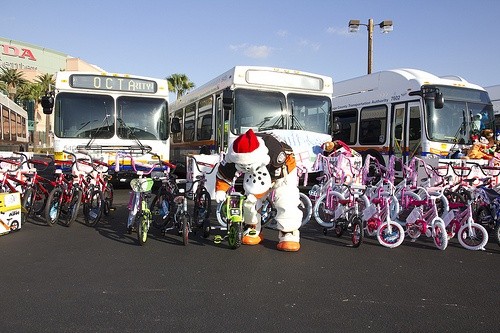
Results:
[320,141,334,152]
[227,129,267,165]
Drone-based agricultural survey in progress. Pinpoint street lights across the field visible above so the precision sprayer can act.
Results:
[347,17,394,74]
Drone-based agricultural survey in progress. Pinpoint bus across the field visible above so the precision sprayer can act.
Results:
[41,70,181,186]
[170,64,343,191]
[0,90,30,153]
[334,68,498,195]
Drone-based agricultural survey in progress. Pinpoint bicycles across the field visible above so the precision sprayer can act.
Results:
[334,183,372,249]
[363,177,406,249]
[12,151,57,224]
[473,186,500,247]
[43,153,74,228]
[212,193,275,250]
[114,152,161,245]
[77,150,117,227]
[146,150,193,247]
[444,185,490,251]
[405,185,450,251]
[62,149,96,227]
[148,155,220,228]
[0,156,49,216]
[215,164,313,230]
[308,149,500,229]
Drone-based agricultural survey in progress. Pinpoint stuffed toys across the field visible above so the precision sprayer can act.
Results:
[214,129,303,252]
[460,129,500,165]
